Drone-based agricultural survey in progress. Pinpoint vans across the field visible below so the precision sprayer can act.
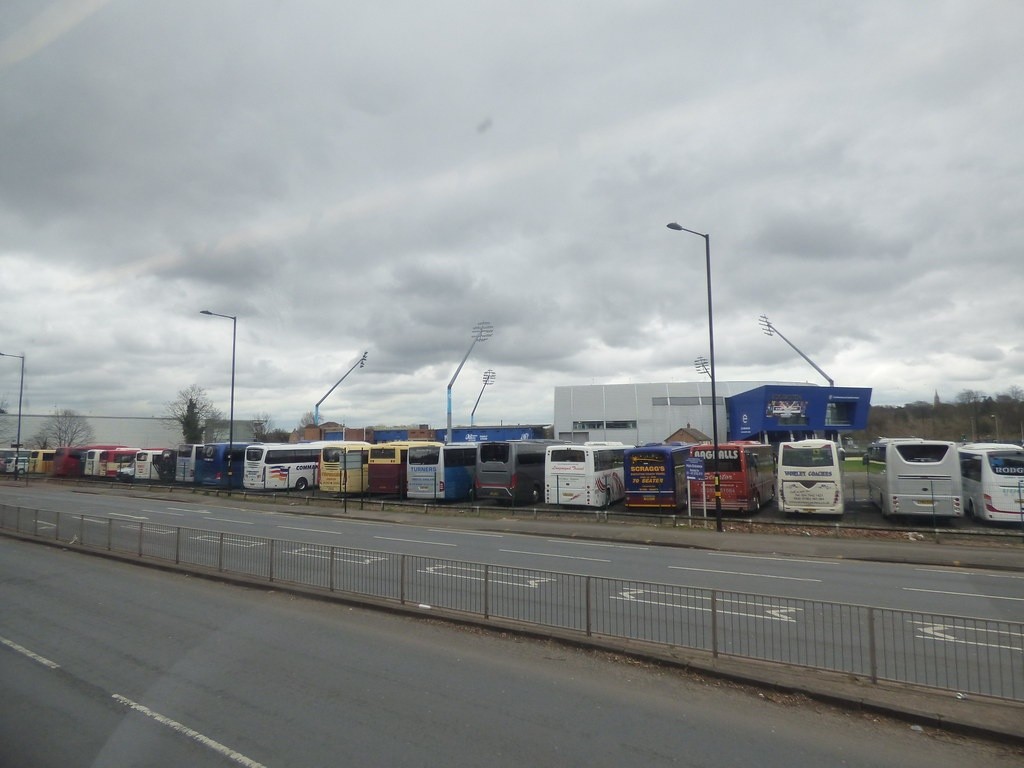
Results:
[6,457,28,475]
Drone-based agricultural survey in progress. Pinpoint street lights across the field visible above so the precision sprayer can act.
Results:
[314,351,368,426]
[0,354,24,447]
[445,322,494,442]
[471,369,496,426]
[666,222,723,532]
[758,314,835,386]
[200,310,236,497]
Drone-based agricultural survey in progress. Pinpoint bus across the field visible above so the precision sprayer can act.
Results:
[0,448,33,472]
[79,447,176,484]
[862,436,964,520]
[175,441,311,490]
[778,439,846,516]
[624,440,777,513]
[406,439,521,503]
[545,442,637,510]
[317,441,443,498]
[957,442,1024,525]
[29,450,56,474]
[475,439,584,506]
[242,441,371,492]
[53,446,128,479]
